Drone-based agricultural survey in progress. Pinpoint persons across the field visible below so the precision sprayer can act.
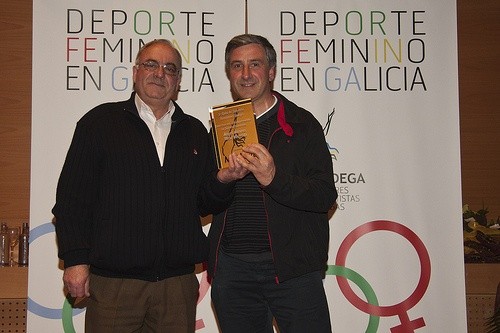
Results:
[51,39,254,333]
[208,34,338,333]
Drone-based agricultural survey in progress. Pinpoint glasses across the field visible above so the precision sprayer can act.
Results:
[138,60,180,75]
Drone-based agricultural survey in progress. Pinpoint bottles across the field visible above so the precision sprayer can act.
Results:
[0,223,9,266]
[19,223,29,267]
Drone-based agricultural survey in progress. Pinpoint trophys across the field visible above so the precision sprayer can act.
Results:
[208,98,260,170]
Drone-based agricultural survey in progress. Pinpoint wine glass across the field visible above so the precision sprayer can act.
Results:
[8,226,19,267]
